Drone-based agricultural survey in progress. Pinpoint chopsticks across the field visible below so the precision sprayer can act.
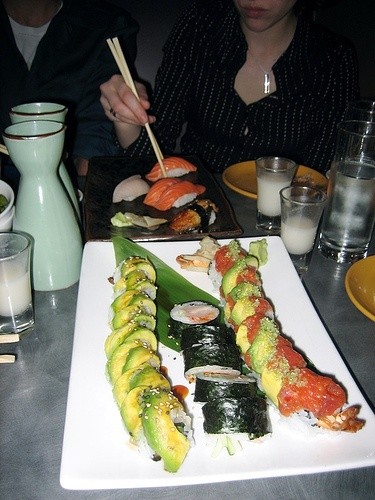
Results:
[0,334,20,362]
[0,144,9,155]
[107,37,167,178]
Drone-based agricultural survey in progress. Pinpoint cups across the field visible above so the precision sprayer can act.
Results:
[0,231,35,333]
[280,185,328,272]
[318,99,375,266]
[256,156,297,236]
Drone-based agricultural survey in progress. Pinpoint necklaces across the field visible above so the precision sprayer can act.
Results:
[249,50,271,94]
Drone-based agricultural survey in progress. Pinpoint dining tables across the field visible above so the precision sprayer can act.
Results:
[0,177,375,500]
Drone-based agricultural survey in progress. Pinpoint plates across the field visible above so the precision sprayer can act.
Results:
[223,160,330,200]
[345,255,375,322]
[325,169,331,180]
[82,154,244,244]
[59,237,375,490]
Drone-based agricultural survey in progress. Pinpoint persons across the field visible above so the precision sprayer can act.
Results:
[0,0,139,176]
[99,0,360,175]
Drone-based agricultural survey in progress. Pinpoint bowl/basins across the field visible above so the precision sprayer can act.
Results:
[0,179,15,233]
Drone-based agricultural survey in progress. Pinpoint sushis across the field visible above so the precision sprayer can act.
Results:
[105,238,364,472]
[112,155,218,231]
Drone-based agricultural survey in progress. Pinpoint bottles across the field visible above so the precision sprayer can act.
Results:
[1,119,84,288]
[10,103,81,227]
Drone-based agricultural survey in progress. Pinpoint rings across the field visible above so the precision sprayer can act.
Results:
[110,108,116,116]
